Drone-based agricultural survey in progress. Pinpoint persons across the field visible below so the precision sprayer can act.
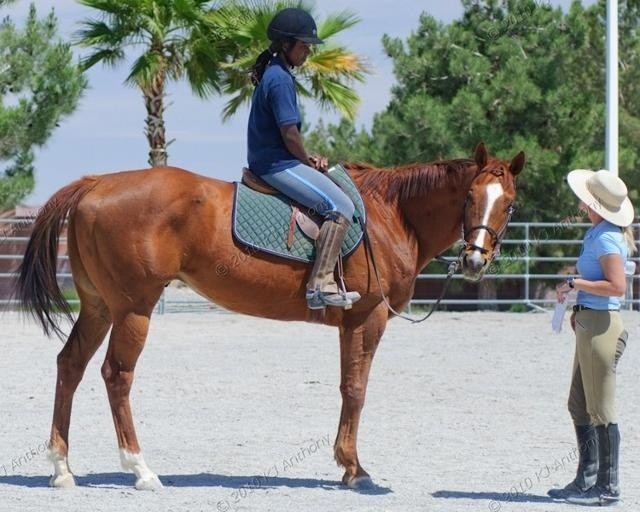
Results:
[547,169,634,503]
[248,5,361,310]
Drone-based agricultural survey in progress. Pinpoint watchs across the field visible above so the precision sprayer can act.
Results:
[566,275,576,291]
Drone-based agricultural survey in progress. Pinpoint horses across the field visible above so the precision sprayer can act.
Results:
[9,139,527,492]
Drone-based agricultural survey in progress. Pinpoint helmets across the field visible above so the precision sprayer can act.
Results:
[268,6,325,46]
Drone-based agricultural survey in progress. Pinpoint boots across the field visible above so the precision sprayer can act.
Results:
[546,421,601,502]
[303,209,362,312]
[565,421,622,506]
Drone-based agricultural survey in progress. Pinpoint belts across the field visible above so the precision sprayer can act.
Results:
[572,303,591,311]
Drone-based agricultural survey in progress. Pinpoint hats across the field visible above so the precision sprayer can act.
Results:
[565,166,637,229]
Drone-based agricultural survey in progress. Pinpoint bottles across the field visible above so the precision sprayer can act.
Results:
[624,260,636,276]
